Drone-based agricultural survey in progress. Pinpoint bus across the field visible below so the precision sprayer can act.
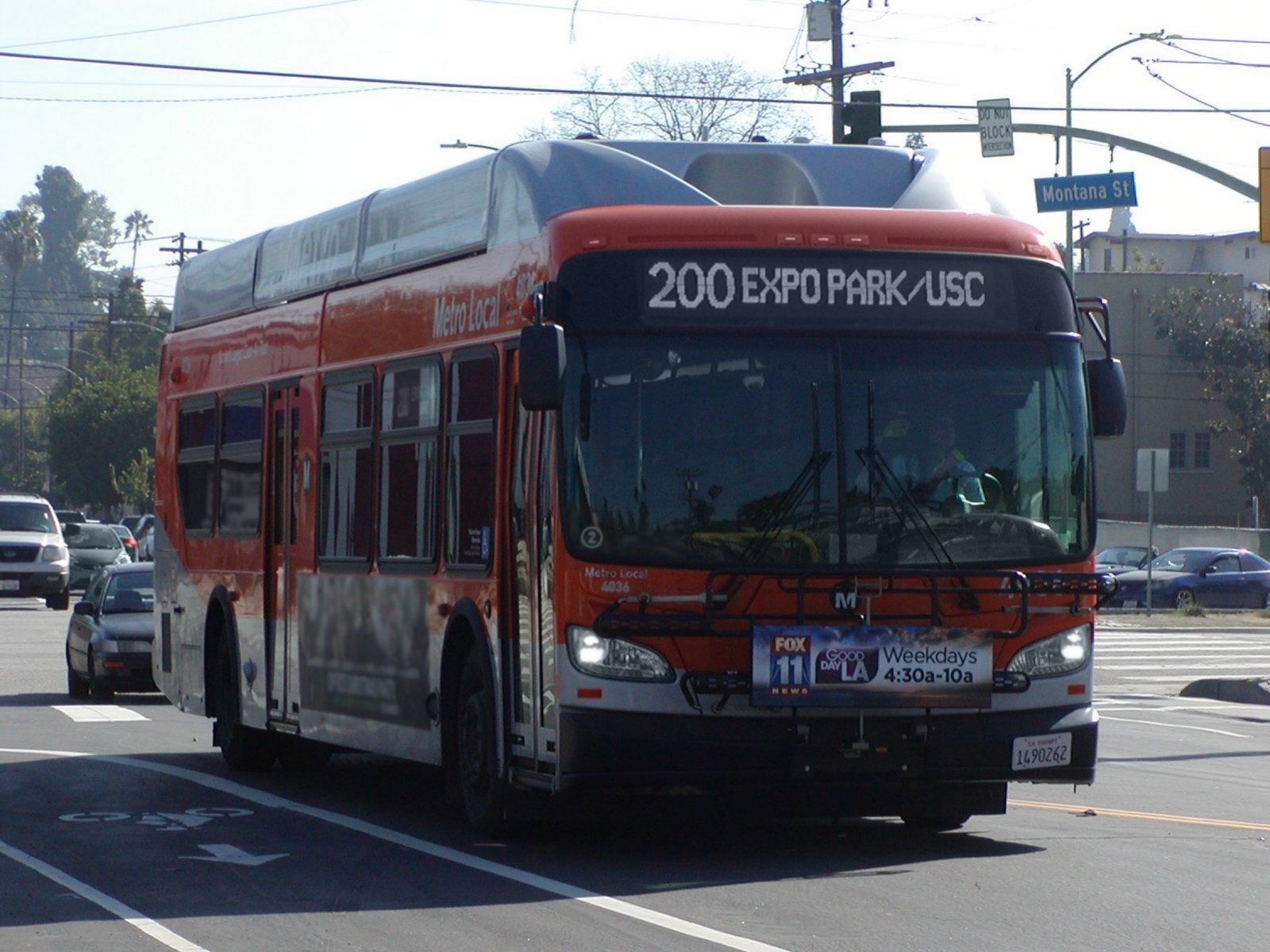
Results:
[150,131,1129,841]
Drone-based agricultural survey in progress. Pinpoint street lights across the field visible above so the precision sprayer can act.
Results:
[1064,30,1184,292]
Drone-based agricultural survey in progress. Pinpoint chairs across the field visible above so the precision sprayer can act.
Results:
[114,591,146,610]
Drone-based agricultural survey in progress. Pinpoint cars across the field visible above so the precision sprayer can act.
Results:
[65,561,161,701]
[41,509,155,595]
[1093,545,1177,609]
[1101,546,1270,612]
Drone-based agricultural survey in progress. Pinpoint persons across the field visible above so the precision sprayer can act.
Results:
[879,410,986,505]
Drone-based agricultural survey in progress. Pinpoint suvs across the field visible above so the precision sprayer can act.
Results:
[0,490,81,611]
